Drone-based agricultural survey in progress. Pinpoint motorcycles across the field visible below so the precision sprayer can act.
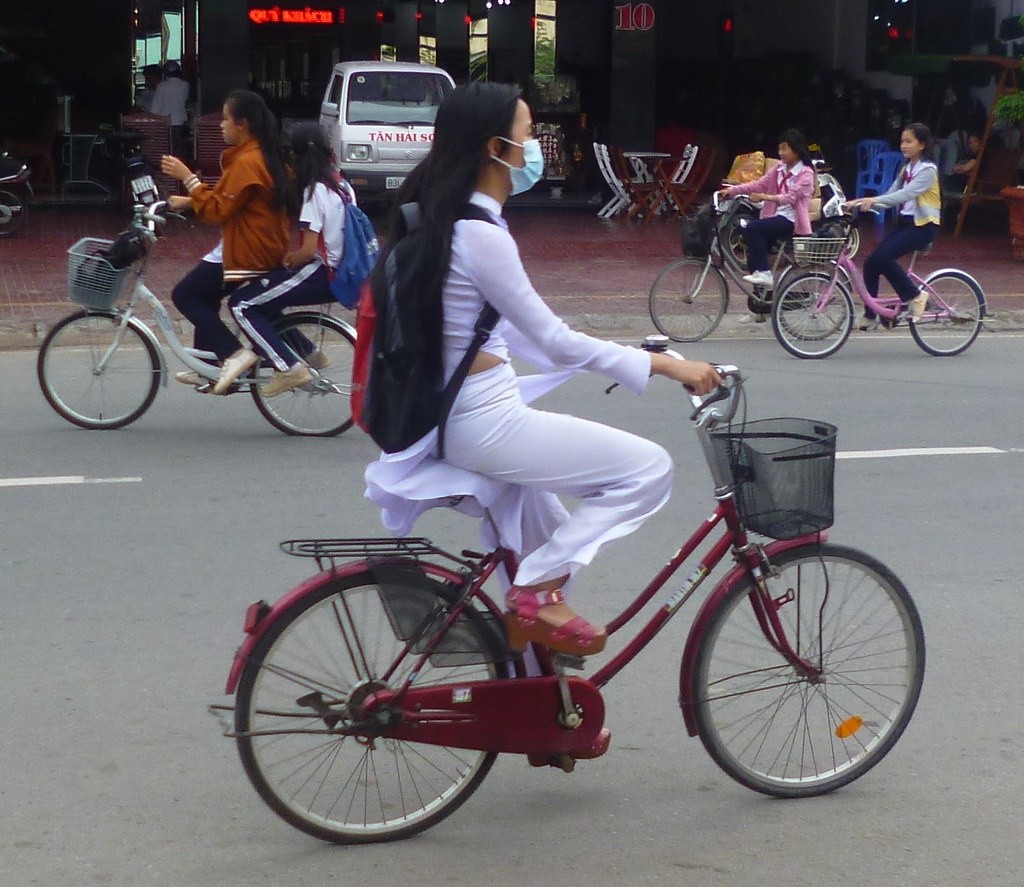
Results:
[107,129,165,236]
[0,156,33,237]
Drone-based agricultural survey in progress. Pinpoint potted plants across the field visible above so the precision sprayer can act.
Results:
[991,91,1024,261]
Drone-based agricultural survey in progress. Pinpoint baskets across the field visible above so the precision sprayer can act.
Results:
[707,416,840,540]
[66,236,133,311]
[792,237,848,268]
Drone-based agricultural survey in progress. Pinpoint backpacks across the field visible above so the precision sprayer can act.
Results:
[350,204,514,454]
[322,178,380,310]
[75,229,146,297]
[682,203,724,268]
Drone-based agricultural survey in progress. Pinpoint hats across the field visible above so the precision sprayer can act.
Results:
[142,64,158,79]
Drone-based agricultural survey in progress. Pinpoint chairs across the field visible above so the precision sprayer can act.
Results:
[398,79,426,105]
[193,111,236,189]
[3,111,59,193]
[852,139,910,224]
[593,142,717,229]
[119,111,180,208]
[972,146,1023,202]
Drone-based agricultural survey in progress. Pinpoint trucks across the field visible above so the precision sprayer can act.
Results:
[281,60,456,201]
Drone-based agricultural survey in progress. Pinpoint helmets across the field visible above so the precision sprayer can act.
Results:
[161,61,180,72]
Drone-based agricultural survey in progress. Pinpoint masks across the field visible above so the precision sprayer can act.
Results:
[487,135,545,196]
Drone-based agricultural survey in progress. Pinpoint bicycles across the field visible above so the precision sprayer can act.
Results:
[771,200,995,361]
[648,188,852,342]
[36,203,359,436]
[208,332,927,847]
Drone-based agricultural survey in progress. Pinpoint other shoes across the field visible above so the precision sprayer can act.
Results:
[851,312,881,329]
[259,365,314,398]
[911,290,930,323]
[307,351,332,370]
[738,311,764,324]
[213,348,259,394]
[176,370,210,387]
[742,270,774,286]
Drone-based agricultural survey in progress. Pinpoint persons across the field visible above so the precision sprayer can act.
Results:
[152,60,189,153]
[842,122,940,331]
[717,126,816,287]
[368,82,722,769]
[135,64,162,108]
[160,91,290,394]
[226,120,357,399]
[943,134,990,192]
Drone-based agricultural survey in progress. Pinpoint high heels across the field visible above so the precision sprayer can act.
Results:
[503,585,607,656]
[528,728,612,767]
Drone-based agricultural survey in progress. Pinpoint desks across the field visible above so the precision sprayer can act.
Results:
[58,134,112,201]
[622,151,671,173]
